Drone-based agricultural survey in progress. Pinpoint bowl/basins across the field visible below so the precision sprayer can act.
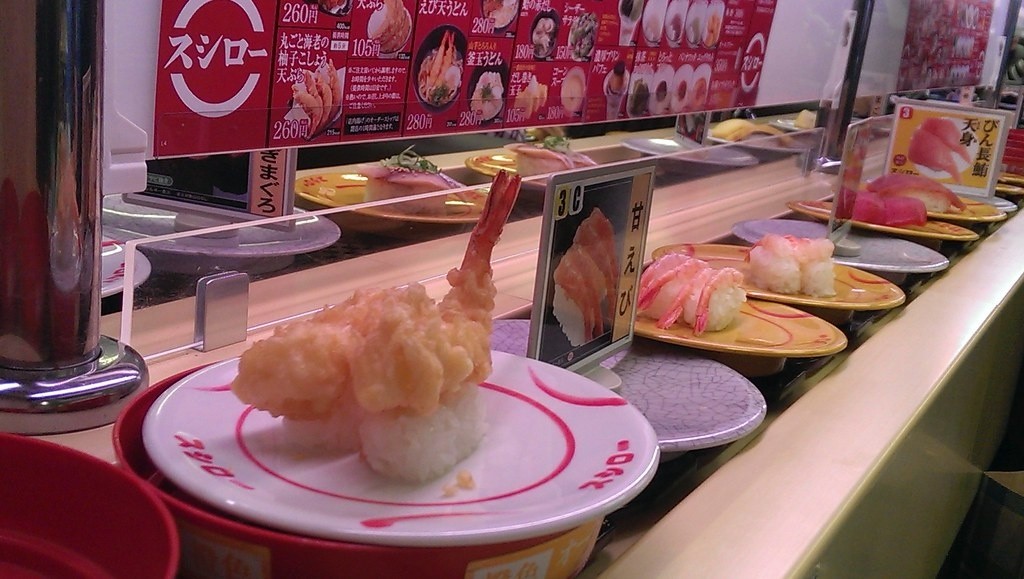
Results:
[480,1,517,33]
[413,24,466,112]
[306,0,352,16]
[529,9,559,60]
[467,56,509,126]
[0,360,601,579]
[368,6,412,58]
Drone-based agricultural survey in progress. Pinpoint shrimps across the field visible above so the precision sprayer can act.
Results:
[553,206,618,342]
[234,170,522,410]
[637,234,834,336]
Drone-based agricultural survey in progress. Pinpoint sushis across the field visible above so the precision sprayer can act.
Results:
[504,135,597,175]
[269,0,1006,146]
[231,170,521,480]
[907,118,972,181]
[358,143,486,216]
[853,171,965,225]
[635,251,747,336]
[553,208,619,345]
[749,234,836,297]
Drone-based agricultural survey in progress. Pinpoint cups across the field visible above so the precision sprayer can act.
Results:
[602,1,724,121]
[514,88,549,119]
[560,65,584,118]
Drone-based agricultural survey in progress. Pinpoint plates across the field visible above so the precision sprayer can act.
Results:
[465,153,548,196]
[100,238,151,297]
[704,129,809,153]
[769,118,820,135]
[620,137,759,166]
[141,183,1020,544]
[103,191,342,274]
[296,173,487,228]
